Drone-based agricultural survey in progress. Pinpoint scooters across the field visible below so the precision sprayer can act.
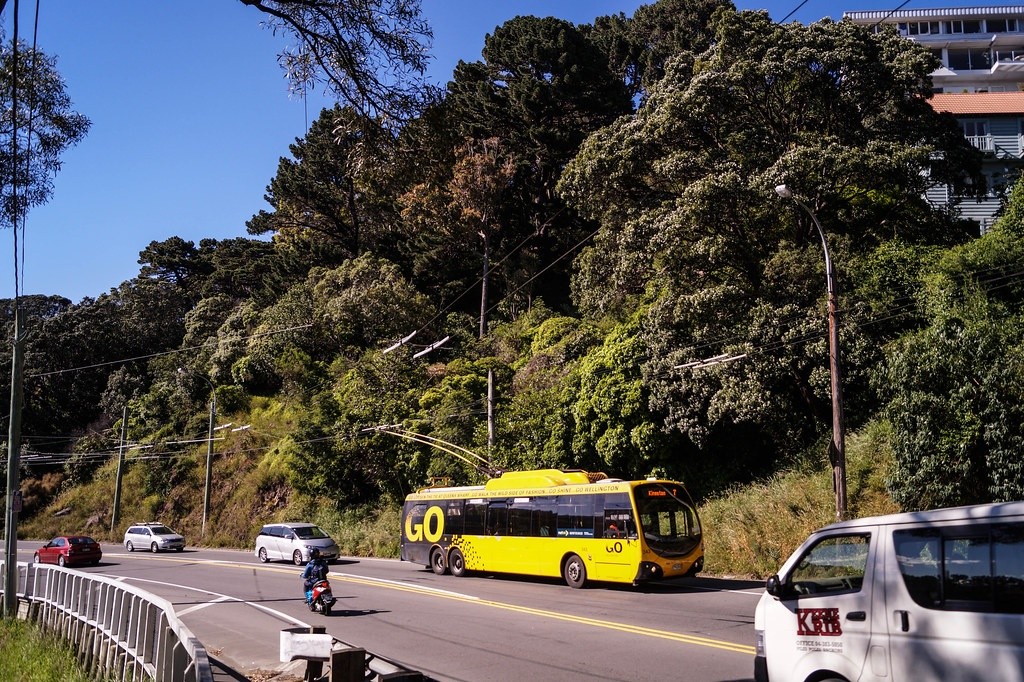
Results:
[301,573,336,616]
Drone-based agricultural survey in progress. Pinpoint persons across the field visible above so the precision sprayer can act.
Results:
[607,516,636,539]
[300,548,329,604]
[647,522,659,537]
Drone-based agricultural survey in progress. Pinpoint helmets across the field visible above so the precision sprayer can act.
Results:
[309,548,319,558]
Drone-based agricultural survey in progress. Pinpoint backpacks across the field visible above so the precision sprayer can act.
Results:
[309,560,324,586]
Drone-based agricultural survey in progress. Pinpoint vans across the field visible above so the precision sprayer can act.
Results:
[753,503,1024,681]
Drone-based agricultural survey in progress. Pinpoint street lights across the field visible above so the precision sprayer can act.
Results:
[776,183,848,525]
[178,367,216,536]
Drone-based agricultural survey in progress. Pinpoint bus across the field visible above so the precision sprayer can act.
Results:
[398,469,705,589]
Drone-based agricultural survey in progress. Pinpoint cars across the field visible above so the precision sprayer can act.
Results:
[123,522,186,554]
[254,523,340,566]
[33,536,103,567]
